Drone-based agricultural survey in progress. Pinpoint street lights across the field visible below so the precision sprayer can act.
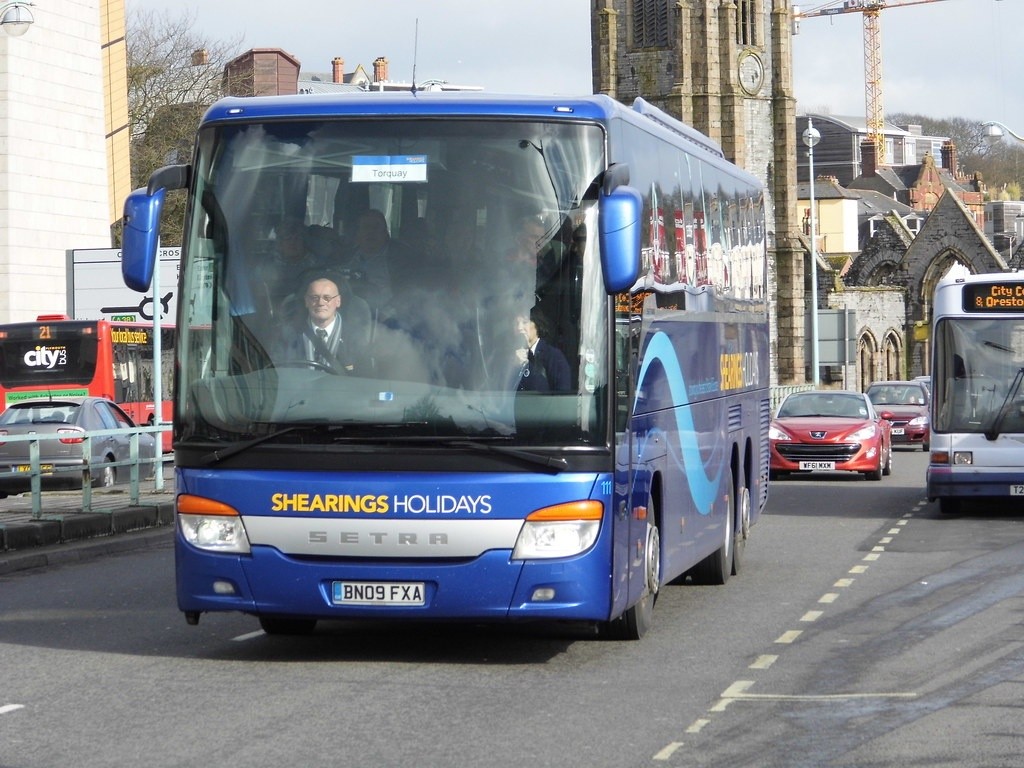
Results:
[803,117,822,384]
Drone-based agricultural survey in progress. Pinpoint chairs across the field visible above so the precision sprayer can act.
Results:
[330,297,373,366]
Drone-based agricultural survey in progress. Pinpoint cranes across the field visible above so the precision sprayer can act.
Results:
[794,0,948,167]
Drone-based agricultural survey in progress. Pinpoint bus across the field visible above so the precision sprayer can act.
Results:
[912,269,1024,514]
[121,78,771,642]
[0,314,212,457]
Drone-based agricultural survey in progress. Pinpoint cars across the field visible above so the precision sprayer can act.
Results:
[769,389,895,482]
[0,395,164,498]
[863,380,932,451]
[900,375,933,415]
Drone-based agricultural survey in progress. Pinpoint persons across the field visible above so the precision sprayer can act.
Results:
[263,217,334,275]
[490,303,571,391]
[495,216,548,273]
[334,208,413,280]
[281,277,367,376]
